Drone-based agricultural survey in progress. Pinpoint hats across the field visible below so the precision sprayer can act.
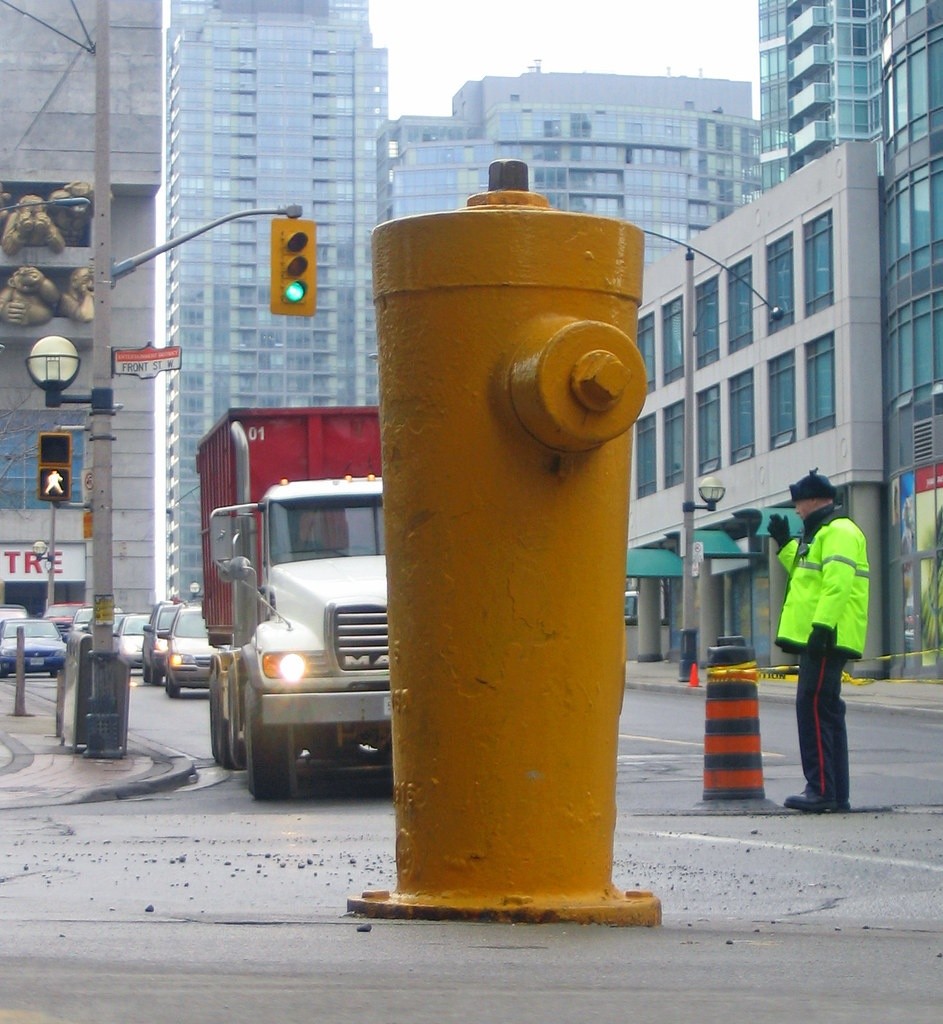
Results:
[789,471,838,503]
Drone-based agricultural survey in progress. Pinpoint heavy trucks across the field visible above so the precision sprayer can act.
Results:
[194,405,392,801]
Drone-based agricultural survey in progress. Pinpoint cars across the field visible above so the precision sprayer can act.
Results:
[64,607,126,633]
[0,604,70,679]
[143,600,183,686]
[157,606,218,698]
[113,615,152,669]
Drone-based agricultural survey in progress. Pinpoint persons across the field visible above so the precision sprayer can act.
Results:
[766,475,869,813]
[0,181,114,327]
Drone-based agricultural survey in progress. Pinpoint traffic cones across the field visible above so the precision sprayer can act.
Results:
[686,663,702,687]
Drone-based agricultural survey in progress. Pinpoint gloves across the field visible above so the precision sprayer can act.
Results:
[807,625,831,659]
[766,514,792,546]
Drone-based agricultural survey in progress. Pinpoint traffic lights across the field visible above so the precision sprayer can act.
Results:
[270,218,317,316]
[37,466,71,500]
[39,432,73,468]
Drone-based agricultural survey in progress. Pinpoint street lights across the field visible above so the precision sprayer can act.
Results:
[26,335,123,758]
[678,475,726,681]
[31,542,56,606]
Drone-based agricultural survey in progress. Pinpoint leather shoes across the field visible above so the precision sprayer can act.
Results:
[785,792,851,812]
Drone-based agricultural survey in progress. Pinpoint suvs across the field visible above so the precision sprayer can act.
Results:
[42,603,92,645]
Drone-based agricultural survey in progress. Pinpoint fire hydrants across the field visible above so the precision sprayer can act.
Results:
[342,160,663,927]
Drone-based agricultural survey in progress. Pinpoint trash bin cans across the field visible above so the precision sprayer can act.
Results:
[58,629,130,755]
